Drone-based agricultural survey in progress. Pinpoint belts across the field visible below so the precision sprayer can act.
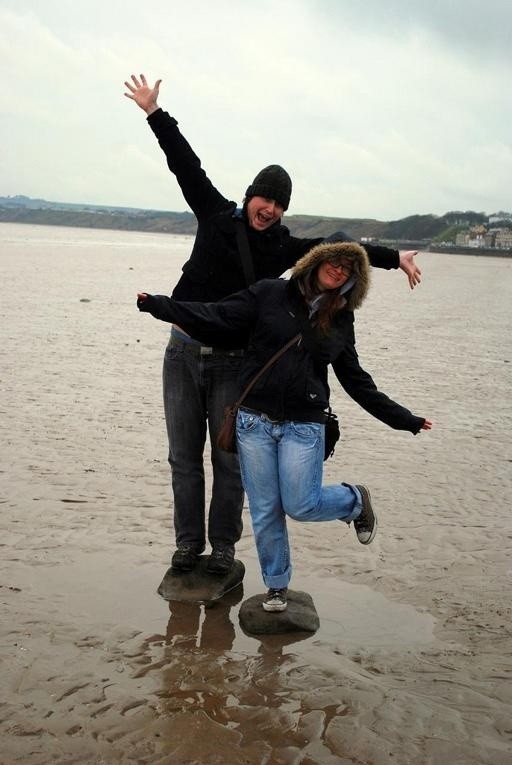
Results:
[240,406,279,423]
[169,335,240,356]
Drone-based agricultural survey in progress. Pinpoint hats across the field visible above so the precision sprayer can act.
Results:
[245,165,292,211]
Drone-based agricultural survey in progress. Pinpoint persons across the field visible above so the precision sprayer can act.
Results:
[124,72,420,588]
[136,230,432,612]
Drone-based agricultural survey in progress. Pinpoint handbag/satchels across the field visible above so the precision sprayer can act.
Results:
[215,405,238,454]
[324,406,340,461]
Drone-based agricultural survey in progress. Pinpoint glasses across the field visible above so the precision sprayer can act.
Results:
[328,259,353,278]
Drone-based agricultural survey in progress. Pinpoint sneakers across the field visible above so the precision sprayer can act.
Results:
[205,542,235,574]
[172,541,205,574]
[262,586,288,611]
[353,484,378,545]
